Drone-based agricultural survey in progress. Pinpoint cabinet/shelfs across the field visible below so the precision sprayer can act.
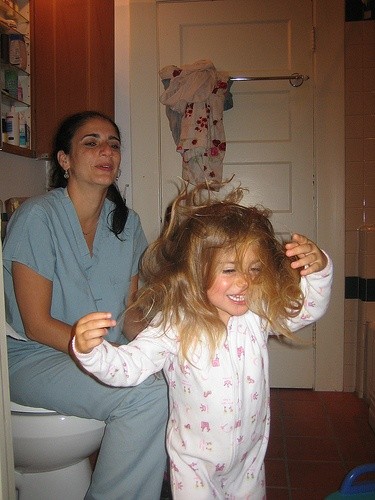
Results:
[0,0,37,159]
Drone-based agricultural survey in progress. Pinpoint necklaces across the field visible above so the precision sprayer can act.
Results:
[82,223,98,236]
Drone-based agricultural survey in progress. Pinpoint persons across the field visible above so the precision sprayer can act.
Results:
[70,170,335,499]
[1,109,171,500]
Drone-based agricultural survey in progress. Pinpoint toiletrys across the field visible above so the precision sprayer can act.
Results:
[0,1,33,154]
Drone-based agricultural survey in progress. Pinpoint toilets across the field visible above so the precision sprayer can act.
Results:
[8,401,108,500]
